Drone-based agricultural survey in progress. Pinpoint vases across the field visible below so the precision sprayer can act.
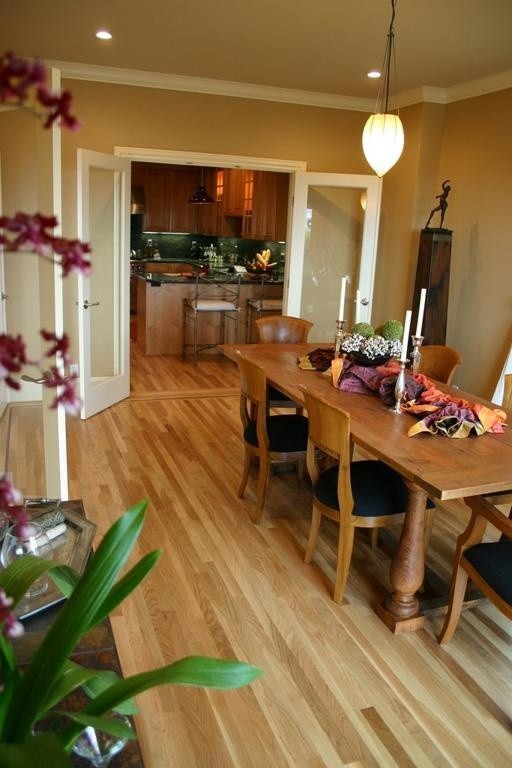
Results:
[340,333,402,360]
[0,46,94,648]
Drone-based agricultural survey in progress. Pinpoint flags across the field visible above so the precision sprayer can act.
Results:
[358,354,384,366]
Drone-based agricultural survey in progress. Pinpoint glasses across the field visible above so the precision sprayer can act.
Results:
[189,167,213,205]
[362,0,405,177]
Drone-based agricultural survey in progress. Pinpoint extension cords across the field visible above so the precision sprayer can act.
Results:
[144,243,154,257]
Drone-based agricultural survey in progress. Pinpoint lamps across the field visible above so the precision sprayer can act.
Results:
[440,492,512,644]
[294,383,436,602]
[234,349,355,523]
[182,273,241,366]
[247,277,283,343]
[251,316,314,420]
[419,345,463,385]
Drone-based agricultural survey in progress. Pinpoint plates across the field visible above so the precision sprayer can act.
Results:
[223,170,277,240]
[143,171,199,234]
[200,168,240,236]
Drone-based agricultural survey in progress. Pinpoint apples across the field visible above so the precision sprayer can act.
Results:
[400,309,412,360]
[339,277,346,321]
[416,288,427,338]
